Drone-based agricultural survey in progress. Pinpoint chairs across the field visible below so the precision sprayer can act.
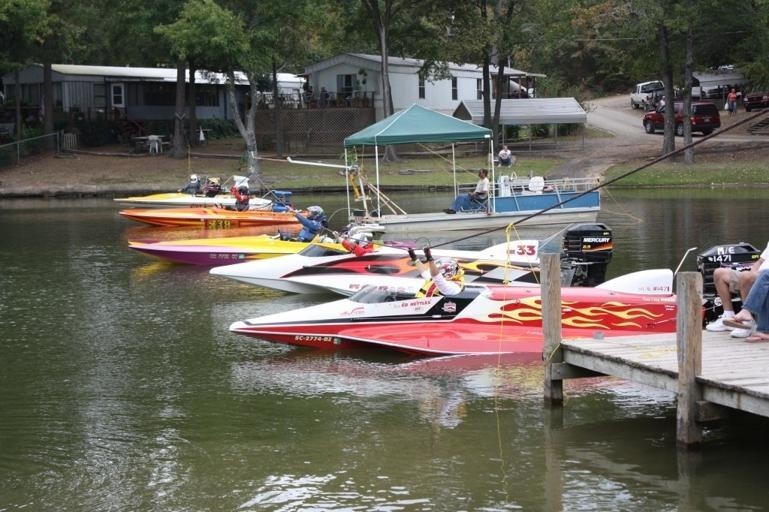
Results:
[474,187,493,212]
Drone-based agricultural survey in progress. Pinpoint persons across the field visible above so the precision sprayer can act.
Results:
[230,185,250,211]
[331,230,374,257]
[408,246,465,297]
[443,169,490,213]
[705,242,768,341]
[203,176,221,196]
[498,145,512,168]
[278,206,326,244]
[727,88,738,118]
[655,95,665,110]
[177,175,201,194]
[302,84,346,107]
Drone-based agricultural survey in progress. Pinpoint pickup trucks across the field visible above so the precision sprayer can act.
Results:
[630,80,665,109]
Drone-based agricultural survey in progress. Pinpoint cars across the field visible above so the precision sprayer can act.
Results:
[744,84,769,112]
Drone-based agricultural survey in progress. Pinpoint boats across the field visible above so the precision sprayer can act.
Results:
[110,176,291,208]
[125,228,385,266]
[334,241,763,369]
[116,205,312,227]
[228,218,615,350]
[231,358,629,430]
[207,237,541,298]
[343,97,600,229]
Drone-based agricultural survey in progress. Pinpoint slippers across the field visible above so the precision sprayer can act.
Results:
[744,331,768,343]
[724,314,750,329]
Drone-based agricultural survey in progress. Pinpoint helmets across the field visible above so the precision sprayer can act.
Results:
[434,258,459,280]
[239,186,248,194]
[190,174,197,183]
[306,206,323,220]
[208,177,218,187]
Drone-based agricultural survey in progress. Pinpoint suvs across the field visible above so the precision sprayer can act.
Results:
[641,102,721,135]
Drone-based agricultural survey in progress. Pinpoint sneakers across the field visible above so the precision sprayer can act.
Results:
[730,320,758,338]
[706,314,735,331]
